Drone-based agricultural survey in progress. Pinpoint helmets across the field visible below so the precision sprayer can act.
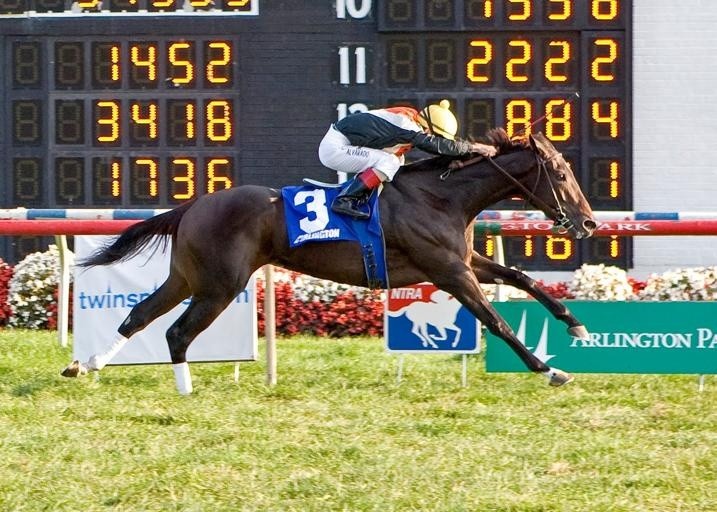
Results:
[418,99,457,141]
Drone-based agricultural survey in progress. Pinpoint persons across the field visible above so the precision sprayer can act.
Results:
[315,100,498,220]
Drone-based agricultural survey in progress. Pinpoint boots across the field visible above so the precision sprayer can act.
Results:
[331,169,381,219]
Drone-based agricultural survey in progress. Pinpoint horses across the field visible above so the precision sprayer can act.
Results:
[60,125,598,397]
